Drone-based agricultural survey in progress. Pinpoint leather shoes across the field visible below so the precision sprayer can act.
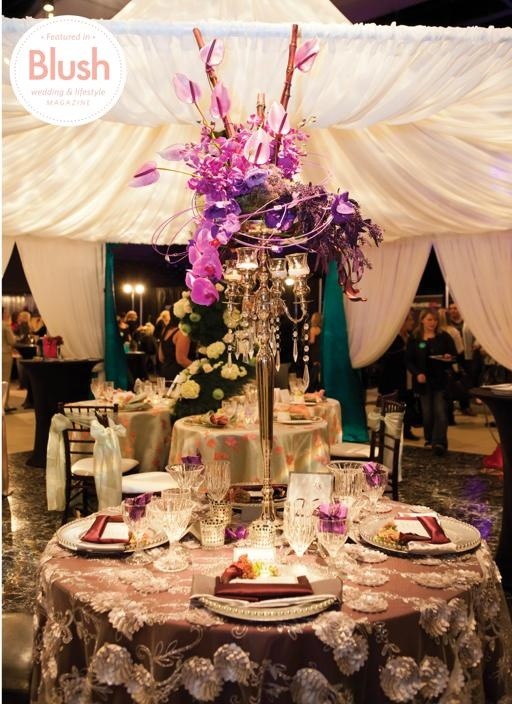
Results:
[403,397,483,456]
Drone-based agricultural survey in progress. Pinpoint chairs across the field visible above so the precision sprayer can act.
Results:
[3,359,511,525]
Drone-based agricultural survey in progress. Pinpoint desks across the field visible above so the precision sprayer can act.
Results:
[30,482,511,704]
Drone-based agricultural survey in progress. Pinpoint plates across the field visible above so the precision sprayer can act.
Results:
[201,562,344,622]
[281,419,312,424]
[58,516,168,554]
[360,511,481,556]
[431,355,456,361]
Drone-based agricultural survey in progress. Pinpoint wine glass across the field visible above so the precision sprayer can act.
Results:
[222,401,237,431]
[120,460,388,572]
[91,377,165,404]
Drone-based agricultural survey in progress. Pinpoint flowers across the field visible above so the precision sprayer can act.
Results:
[130,24,384,306]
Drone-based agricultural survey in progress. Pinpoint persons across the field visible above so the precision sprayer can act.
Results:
[372,300,506,454]
[1,306,193,413]
